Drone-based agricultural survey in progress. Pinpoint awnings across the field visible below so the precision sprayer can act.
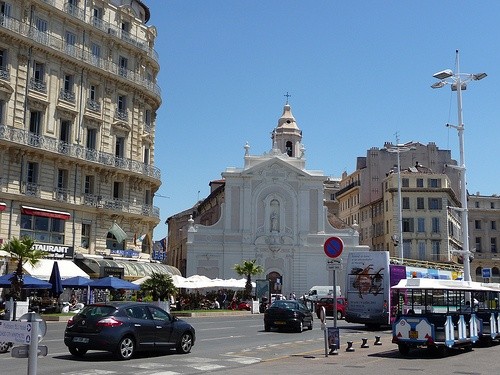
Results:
[0,202,7,210]
[21,205,70,220]
[85,259,183,280]
[22,259,90,280]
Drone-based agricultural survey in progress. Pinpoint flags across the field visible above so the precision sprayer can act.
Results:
[137,233,146,242]
[133,231,138,245]
[109,222,126,243]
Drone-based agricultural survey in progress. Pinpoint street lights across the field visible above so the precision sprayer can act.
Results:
[431,50,488,308]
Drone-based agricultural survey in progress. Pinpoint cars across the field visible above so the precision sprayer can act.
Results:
[264,300,314,333]
[271,294,286,302]
[64,301,196,361]
[316,297,345,320]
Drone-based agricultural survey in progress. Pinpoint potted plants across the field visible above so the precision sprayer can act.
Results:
[235,259,265,314]
[140,271,179,314]
[1,234,50,322]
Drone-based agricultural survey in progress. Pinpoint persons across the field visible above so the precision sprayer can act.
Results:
[177,291,215,310]
[217,289,252,310]
[276,292,307,307]
[69,294,79,309]
[271,205,280,231]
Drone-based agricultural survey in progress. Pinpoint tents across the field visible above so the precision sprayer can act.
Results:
[80,275,140,305]
[62,276,94,304]
[0,273,52,298]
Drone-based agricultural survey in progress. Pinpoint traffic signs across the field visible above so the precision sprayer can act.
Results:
[0,320,33,345]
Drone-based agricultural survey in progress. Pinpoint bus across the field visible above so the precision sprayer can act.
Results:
[344,251,473,330]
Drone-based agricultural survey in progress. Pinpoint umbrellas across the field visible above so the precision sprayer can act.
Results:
[131,276,151,285]
[169,274,215,288]
[49,261,63,298]
[211,278,257,288]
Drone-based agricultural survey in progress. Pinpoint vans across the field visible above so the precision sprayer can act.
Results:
[300,285,342,301]
